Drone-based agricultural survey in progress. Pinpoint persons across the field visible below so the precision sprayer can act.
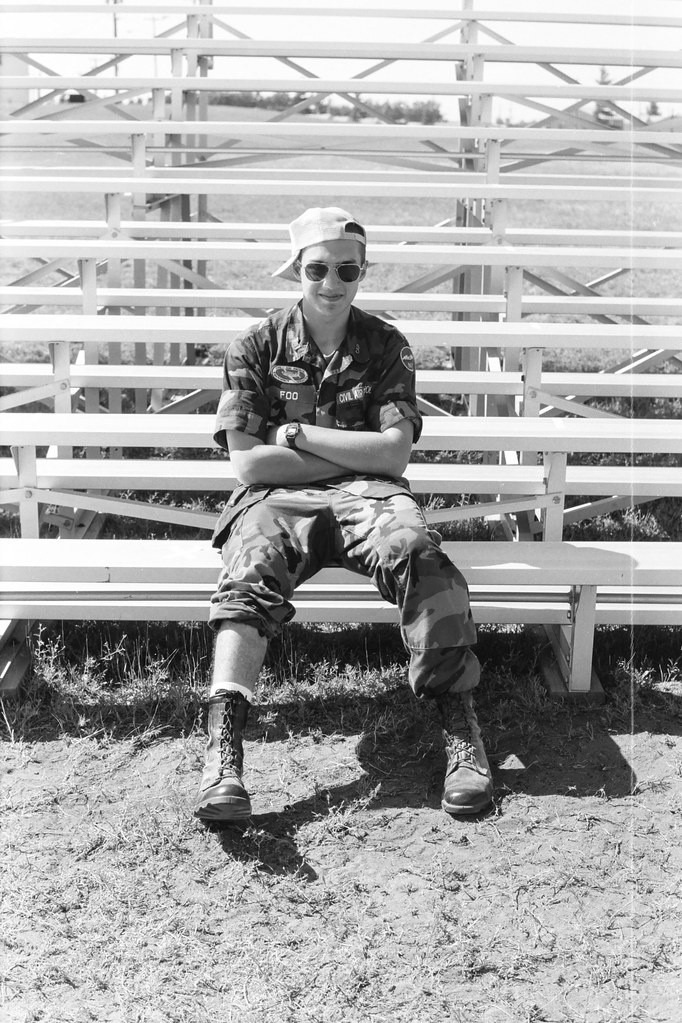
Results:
[192,206,494,821]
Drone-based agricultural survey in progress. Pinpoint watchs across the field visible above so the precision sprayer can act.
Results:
[285,420,300,450]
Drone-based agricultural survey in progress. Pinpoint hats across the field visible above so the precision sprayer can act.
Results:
[270,207,366,282]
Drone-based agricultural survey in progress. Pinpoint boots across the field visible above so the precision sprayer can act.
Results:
[194,688,252,820]
[434,690,493,813]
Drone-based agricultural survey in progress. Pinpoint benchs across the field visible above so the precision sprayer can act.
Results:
[0,43,682,697]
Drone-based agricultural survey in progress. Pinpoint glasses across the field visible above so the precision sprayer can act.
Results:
[298,261,363,283]
